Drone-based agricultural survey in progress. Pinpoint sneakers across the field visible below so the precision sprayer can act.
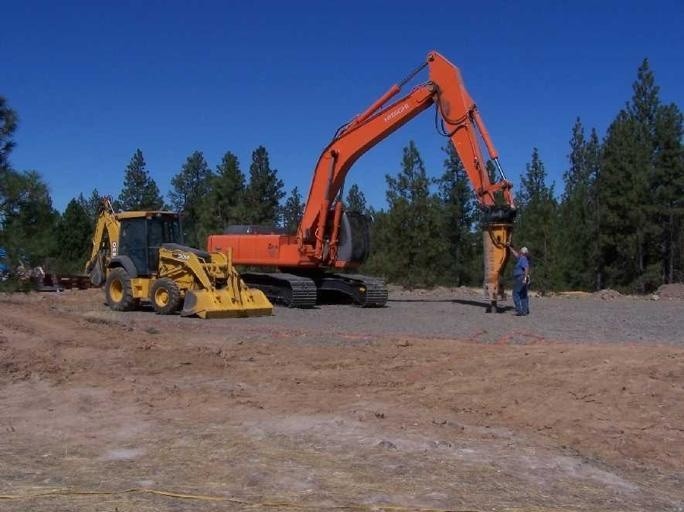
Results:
[512,310,529,316]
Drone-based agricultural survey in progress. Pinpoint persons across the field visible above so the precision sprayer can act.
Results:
[506,241,530,316]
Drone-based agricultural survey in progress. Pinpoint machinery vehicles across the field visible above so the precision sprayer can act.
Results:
[207,49,518,313]
[85,198,273,319]
